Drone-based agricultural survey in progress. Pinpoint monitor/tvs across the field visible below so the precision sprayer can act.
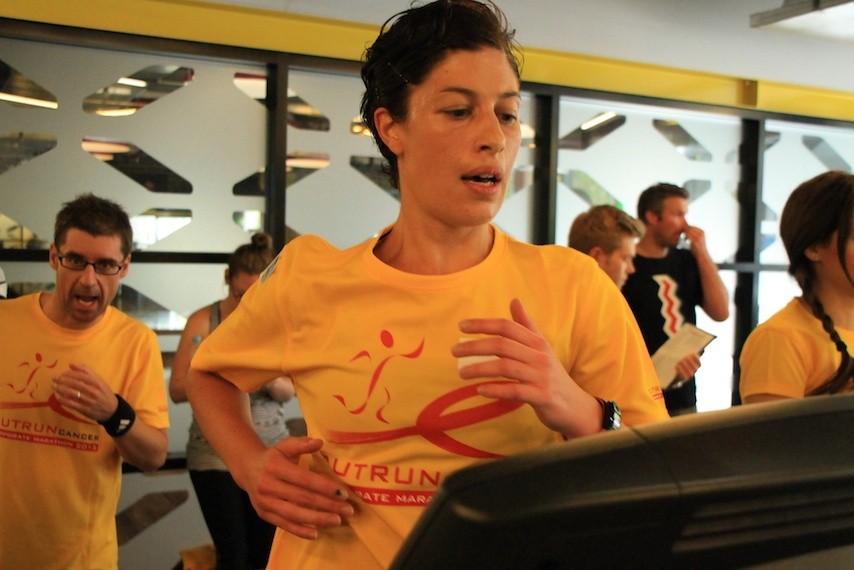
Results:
[391,391,854,570]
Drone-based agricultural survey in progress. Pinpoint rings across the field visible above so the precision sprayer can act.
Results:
[77,391,80,401]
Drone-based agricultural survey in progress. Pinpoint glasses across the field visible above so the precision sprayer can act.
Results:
[55,245,127,276]
[229,280,245,302]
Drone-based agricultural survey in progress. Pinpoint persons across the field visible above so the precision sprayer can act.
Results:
[738,170,854,406]
[168,230,294,570]
[620,180,730,421]
[565,204,702,430]
[1,188,171,570]
[181,0,669,570]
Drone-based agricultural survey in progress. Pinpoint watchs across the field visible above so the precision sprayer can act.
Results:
[562,394,621,444]
